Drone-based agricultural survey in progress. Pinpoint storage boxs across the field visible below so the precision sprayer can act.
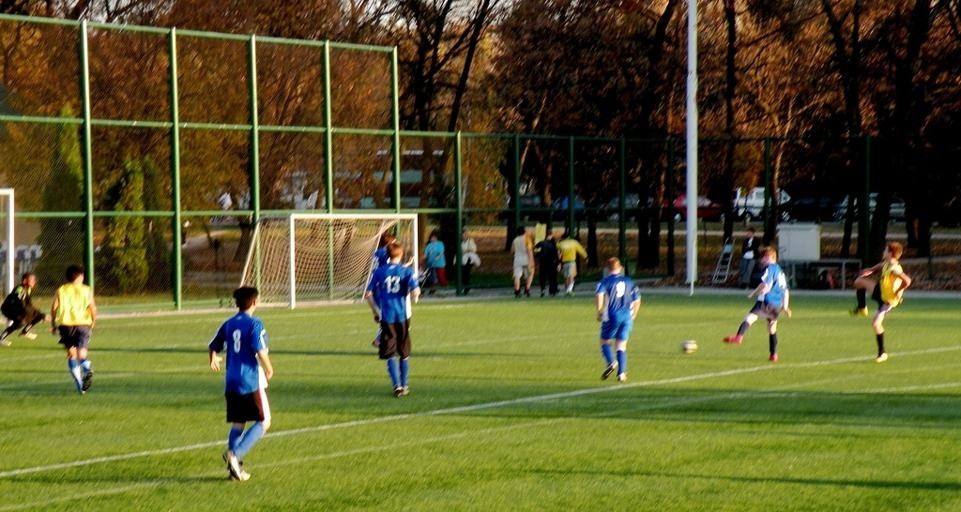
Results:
[775,224,823,262]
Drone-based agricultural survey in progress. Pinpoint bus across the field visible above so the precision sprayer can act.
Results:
[263,142,478,222]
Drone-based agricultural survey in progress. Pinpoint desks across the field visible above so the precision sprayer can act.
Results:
[780,259,860,289]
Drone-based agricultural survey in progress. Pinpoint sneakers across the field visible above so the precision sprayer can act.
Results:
[722,334,744,344]
[875,352,888,363]
[600,360,618,381]
[850,307,870,318]
[230,471,251,481]
[77,369,94,395]
[0,338,14,346]
[428,287,474,299]
[514,289,576,298]
[392,383,410,398]
[769,353,779,362]
[617,374,628,382]
[223,450,242,482]
[18,332,39,340]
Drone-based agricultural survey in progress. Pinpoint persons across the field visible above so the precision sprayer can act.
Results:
[533,231,562,297]
[50,263,97,396]
[208,287,275,483]
[0,272,45,347]
[368,234,415,348]
[594,255,642,384]
[364,242,423,398]
[849,240,912,361]
[510,224,536,298]
[420,229,449,295]
[738,228,762,287]
[722,244,795,363]
[555,233,590,296]
[453,226,483,295]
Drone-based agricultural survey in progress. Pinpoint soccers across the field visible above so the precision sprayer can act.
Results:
[681,340,697,354]
